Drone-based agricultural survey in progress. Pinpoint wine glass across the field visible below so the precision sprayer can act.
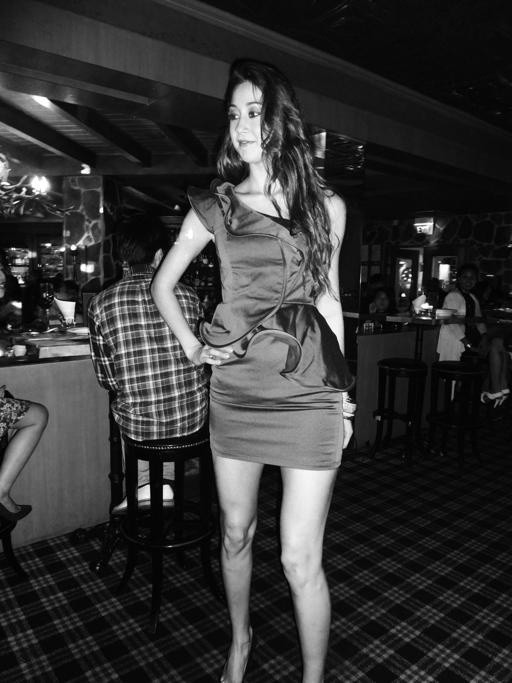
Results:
[40,282,56,333]
[5,245,66,290]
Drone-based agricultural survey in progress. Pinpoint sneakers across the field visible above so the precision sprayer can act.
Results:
[107,482,177,518]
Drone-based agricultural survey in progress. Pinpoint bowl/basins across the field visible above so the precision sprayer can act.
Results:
[434,307,460,317]
[53,298,77,320]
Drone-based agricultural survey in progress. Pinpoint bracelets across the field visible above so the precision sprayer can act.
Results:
[342,394,358,420]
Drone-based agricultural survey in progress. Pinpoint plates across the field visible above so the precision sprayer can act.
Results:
[67,326,90,334]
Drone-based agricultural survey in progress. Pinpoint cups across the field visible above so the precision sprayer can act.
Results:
[189,252,215,317]
[14,344,28,355]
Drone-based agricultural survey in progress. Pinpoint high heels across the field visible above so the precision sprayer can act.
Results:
[498,388,510,407]
[480,390,504,408]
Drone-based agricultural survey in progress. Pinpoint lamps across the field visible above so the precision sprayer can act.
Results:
[0,153,78,221]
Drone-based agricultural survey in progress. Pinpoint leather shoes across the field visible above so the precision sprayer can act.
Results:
[217,624,256,683]
[0,502,33,521]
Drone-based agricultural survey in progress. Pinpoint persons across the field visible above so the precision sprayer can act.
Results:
[147,58,361,683]
[422,276,448,312]
[0,253,50,522]
[437,261,490,423]
[367,287,391,315]
[87,211,215,518]
[482,317,511,405]
[366,271,389,290]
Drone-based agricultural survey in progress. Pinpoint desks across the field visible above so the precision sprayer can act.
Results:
[384,315,482,360]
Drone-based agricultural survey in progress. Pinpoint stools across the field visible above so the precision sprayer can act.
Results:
[114,435,224,637]
[0,431,28,579]
[424,359,486,466]
[367,357,430,467]
